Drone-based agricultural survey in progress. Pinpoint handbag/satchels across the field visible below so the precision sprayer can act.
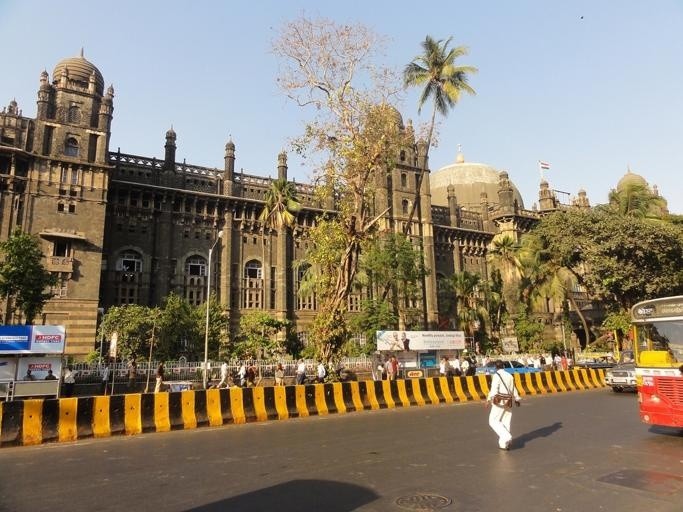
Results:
[493,394,512,408]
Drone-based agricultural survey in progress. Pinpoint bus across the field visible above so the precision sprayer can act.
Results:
[629,295,682,437]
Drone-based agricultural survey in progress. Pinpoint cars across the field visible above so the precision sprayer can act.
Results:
[566,356,618,369]
[604,350,637,394]
[476,359,543,374]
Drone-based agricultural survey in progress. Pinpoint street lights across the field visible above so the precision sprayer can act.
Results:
[202,230,225,390]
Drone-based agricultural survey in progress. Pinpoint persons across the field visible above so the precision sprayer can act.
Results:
[154,359,163,392]
[388,332,404,349]
[483,360,523,449]
[128,361,137,393]
[401,331,410,350]
[63,364,78,397]
[98,362,115,394]
[44,369,55,380]
[22,369,34,380]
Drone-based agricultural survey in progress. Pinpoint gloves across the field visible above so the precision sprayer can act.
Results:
[515,400,520,407]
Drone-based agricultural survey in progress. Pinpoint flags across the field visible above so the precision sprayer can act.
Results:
[539,160,550,169]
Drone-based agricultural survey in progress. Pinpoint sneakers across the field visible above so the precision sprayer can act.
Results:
[499,440,511,451]
